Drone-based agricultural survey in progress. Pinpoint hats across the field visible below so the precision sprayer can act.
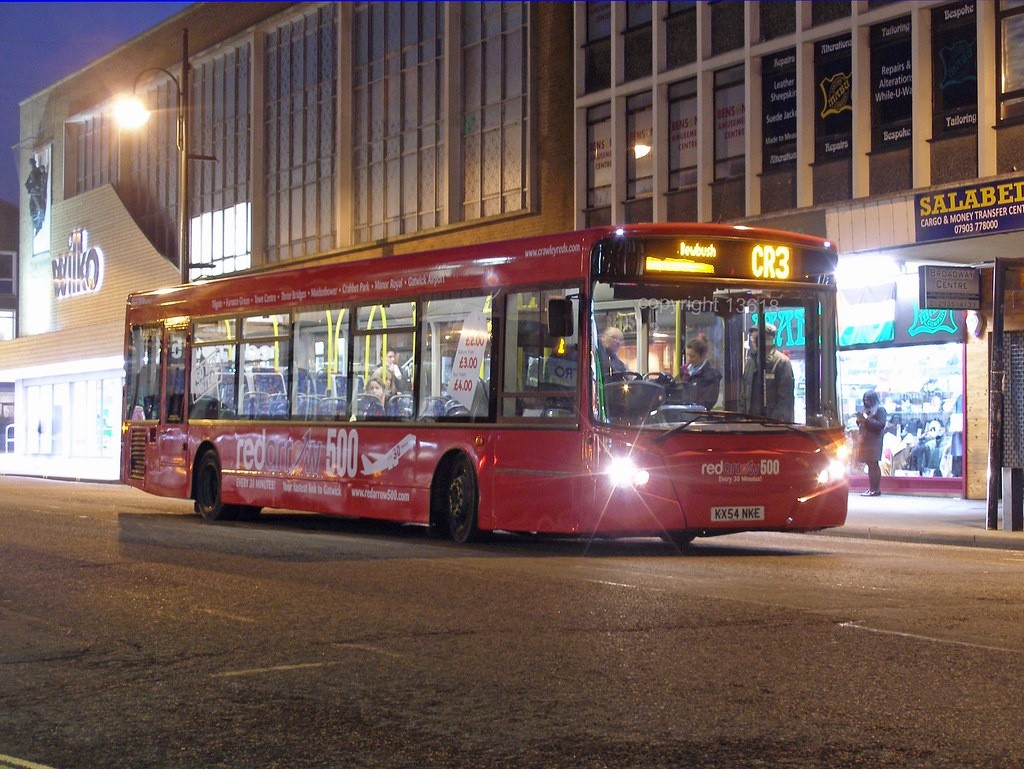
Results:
[750,324,777,337]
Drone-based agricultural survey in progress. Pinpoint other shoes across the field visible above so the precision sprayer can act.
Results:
[862,489,882,495]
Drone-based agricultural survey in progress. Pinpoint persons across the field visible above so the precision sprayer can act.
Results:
[25,157,46,230]
[363,346,410,417]
[594,323,793,422]
[890,390,963,477]
[855,390,887,496]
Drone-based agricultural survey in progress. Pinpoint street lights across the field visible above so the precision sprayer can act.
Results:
[116,27,189,282]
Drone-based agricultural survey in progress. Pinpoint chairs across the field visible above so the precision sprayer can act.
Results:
[599,367,701,414]
[161,361,477,420]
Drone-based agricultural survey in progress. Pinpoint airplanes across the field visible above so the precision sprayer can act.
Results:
[360,433,416,476]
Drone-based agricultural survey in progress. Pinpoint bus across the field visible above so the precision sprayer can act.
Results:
[119,223,849,546]
